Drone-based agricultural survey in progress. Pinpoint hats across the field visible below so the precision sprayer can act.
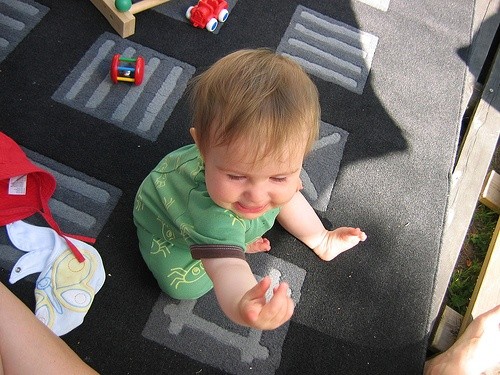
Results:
[0,131,56,226]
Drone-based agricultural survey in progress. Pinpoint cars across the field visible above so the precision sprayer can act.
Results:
[185,0,229,32]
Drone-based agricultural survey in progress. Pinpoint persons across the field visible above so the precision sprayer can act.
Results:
[0,279,107,374]
[133,47,368,331]
